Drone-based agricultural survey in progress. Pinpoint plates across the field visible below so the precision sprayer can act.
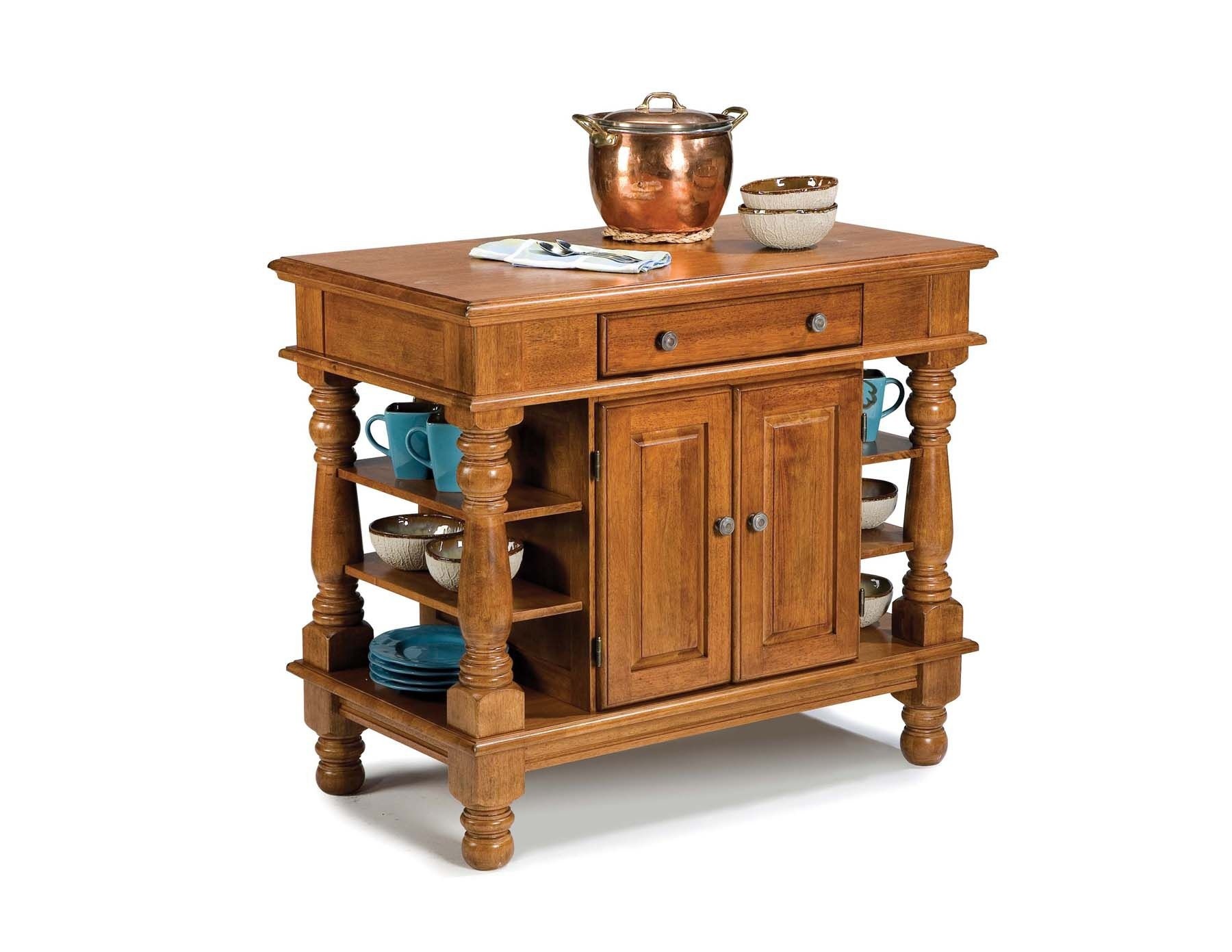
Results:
[368,624,510,692]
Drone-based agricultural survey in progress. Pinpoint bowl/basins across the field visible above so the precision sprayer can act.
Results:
[859,573,893,627]
[368,514,465,571]
[861,479,898,530]
[738,174,839,250]
[424,534,524,594]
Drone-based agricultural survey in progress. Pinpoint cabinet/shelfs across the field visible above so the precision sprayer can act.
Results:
[264,211,998,872]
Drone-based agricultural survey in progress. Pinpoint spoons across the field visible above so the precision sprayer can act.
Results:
[536,239,642,263]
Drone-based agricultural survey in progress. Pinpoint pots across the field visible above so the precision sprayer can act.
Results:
[572,91,748,234]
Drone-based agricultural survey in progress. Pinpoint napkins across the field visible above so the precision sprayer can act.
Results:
[468,238,673,273]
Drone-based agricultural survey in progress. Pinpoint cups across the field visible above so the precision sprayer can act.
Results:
[365,402,464,492]
[862,369,904,442]
